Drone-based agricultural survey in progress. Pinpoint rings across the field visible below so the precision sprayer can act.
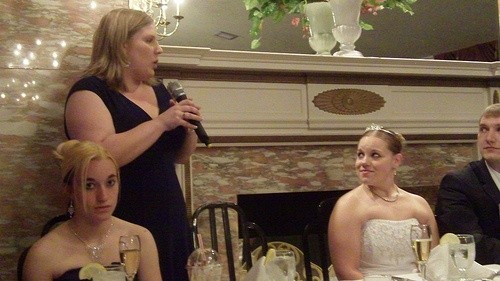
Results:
[182,111,187,118]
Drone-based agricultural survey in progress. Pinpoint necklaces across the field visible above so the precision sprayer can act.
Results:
[368,182,401,203]
[66,216,117,257]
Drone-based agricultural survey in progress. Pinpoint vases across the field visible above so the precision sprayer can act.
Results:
[245,0,336,55]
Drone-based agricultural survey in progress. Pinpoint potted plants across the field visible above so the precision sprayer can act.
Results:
[323,0,416,57]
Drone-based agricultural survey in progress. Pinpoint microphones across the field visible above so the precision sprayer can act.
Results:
[167,79,210,146]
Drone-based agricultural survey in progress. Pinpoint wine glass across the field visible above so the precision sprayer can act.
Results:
[449,233,477,281]
[119,234,143,281]
[410,224,431,281]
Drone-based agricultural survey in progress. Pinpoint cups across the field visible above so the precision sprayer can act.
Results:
[185,248,224,281]
[93,268,126,281]
[274,250,297,281]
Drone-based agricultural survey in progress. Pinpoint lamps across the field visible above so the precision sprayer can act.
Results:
[135,0,184,40]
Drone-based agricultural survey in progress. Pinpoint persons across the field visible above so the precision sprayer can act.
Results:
[326,121,443,281]
[22,139,161,281]
[436,101,500,269]
[63,8,201,280]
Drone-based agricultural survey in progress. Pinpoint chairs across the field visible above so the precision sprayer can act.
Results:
[303,197,339,281]
[193,201,267,281]
